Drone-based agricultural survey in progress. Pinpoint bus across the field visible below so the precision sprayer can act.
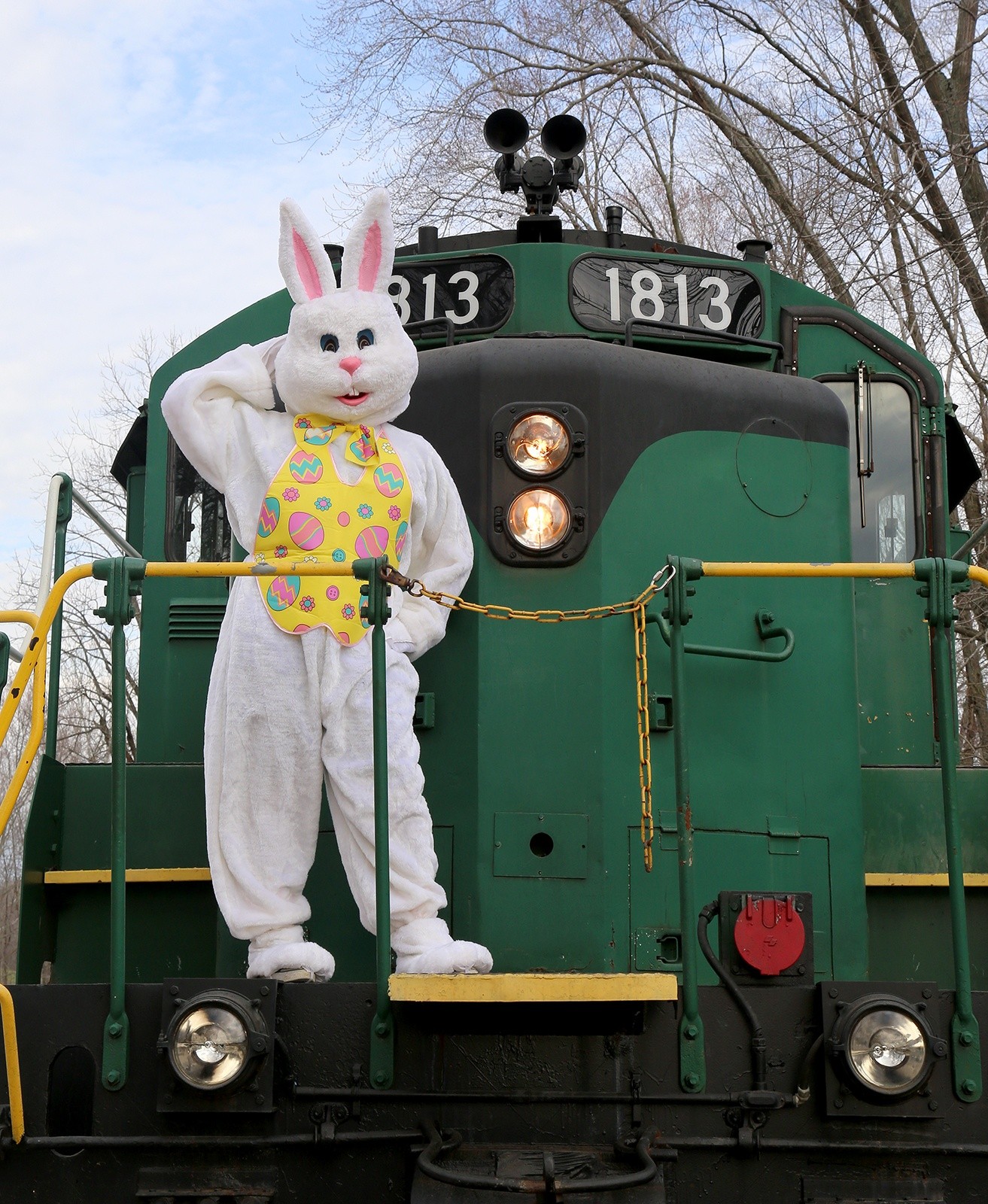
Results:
[0,111,988,1204]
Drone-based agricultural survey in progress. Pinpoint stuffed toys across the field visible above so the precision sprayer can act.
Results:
[160,189,498,981]
